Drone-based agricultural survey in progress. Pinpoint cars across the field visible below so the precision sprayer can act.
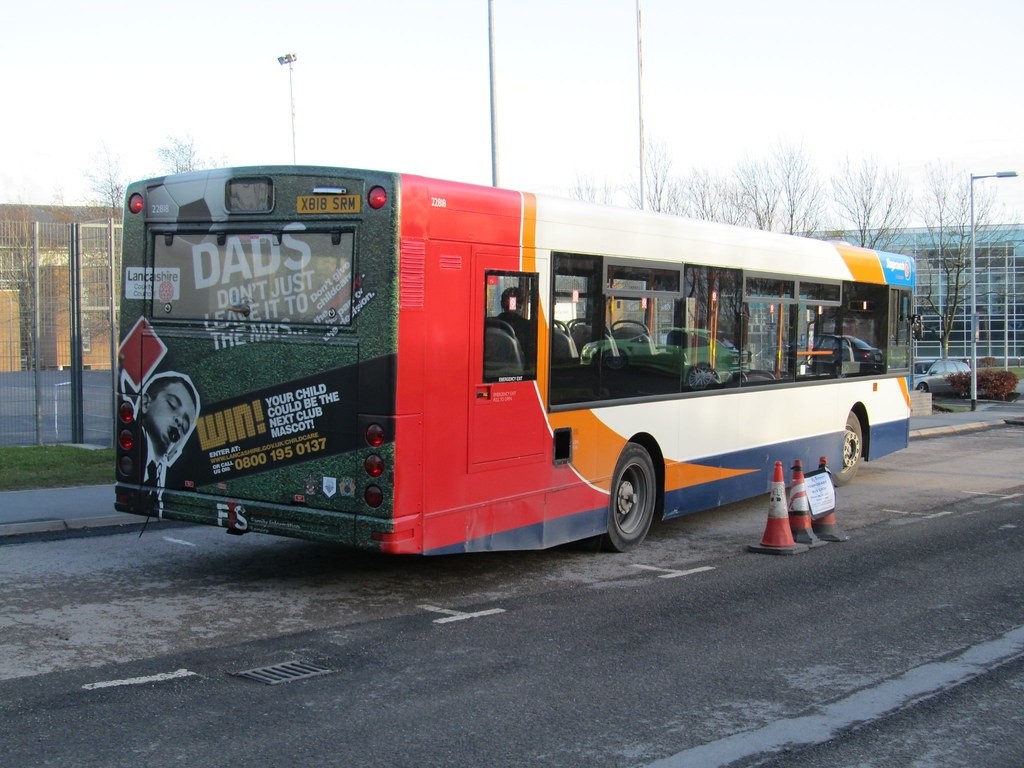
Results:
[755,333,884,378]
[580,328,750,391]
[915,360,971,394]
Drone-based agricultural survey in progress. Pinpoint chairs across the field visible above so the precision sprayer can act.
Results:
[485,318,650,381]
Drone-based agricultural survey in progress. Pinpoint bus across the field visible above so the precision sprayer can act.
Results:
[113,165,924,555]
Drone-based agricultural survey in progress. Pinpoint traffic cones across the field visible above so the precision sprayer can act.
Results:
[747,461,809,555]
[811,457,851,542]
[788,460,828,548]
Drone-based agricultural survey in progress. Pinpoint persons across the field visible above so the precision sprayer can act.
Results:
[490,287,530,363]
[136,375,197,491]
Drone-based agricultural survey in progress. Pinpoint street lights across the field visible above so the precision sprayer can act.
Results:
[279,53,297,165]
[970,171,1019,412]
